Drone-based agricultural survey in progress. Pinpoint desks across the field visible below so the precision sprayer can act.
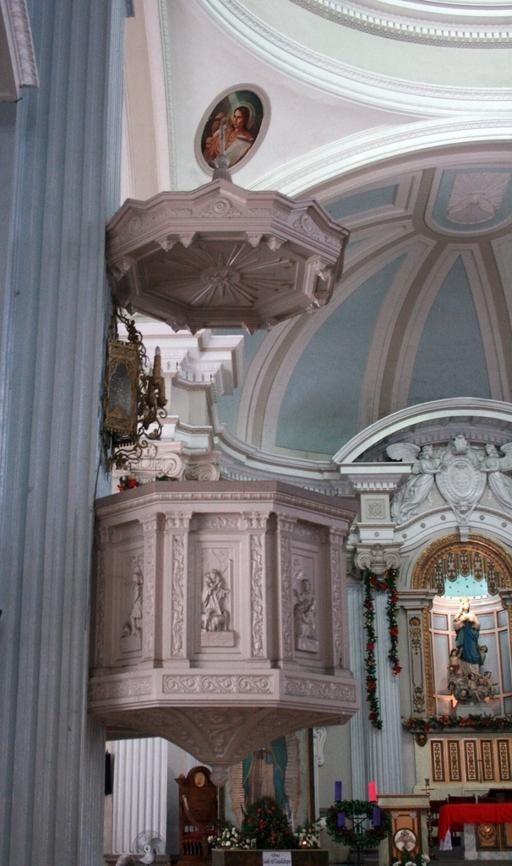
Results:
[223,849,328,866]
[437,803,512,861]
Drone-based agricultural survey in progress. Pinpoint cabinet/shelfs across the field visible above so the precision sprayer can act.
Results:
[173,765,218,866]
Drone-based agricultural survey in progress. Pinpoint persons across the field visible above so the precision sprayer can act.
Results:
[292,579,319,638]
[396,444,445,520]
[203,101,252,160]
[129,571,144,636]
[477,438,512,509]
[201,569,229,634]
[446,598,496,703]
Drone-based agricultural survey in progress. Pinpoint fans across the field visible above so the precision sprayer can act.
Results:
[131,829,170,865]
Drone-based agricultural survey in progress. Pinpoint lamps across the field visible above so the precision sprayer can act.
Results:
[99,302,168,471]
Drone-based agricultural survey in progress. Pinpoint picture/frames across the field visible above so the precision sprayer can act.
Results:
[193,82,271,179]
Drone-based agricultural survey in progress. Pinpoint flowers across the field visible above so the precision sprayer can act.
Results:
[242,796,299,850]
[363,566,403,730]
[401,712,511,735]
[116,475,140,490]
[207,825,250,850]
[322,798,395,849]
